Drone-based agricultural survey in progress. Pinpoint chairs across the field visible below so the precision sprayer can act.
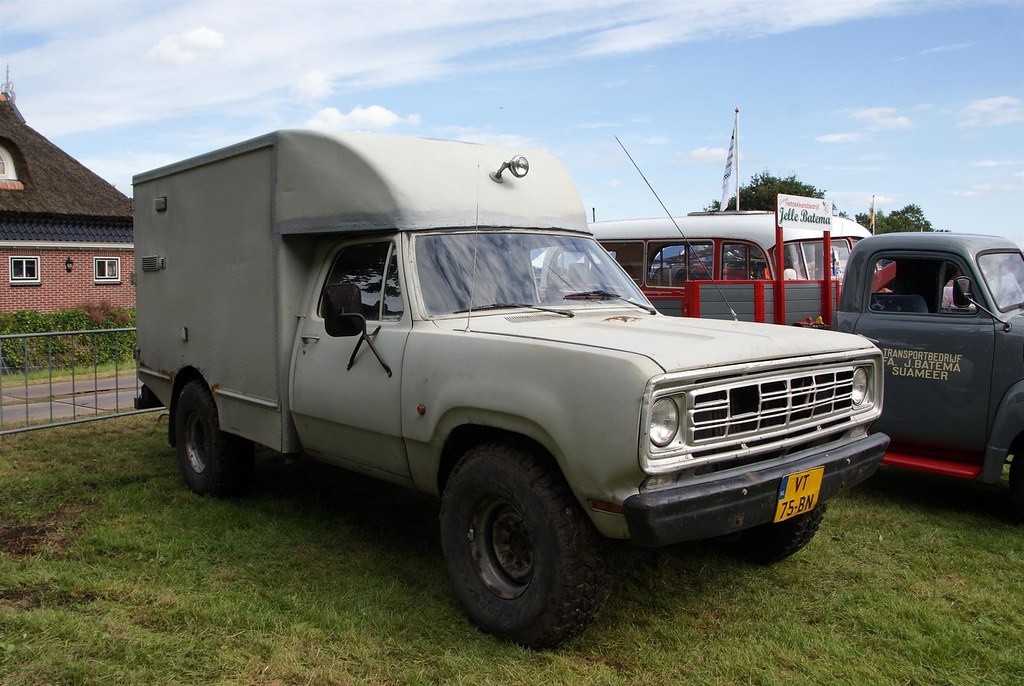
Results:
[653,267,702,286]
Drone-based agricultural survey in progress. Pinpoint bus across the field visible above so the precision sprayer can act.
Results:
[537,209,875,300]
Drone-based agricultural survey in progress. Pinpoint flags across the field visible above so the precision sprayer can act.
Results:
[718,118,738,212]
[866,197,875,228]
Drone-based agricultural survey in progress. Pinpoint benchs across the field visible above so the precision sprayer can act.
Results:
[871,294,929,312]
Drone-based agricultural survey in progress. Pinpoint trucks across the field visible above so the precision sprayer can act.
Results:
[130,126,892,646]
[642,231,1024,521]
